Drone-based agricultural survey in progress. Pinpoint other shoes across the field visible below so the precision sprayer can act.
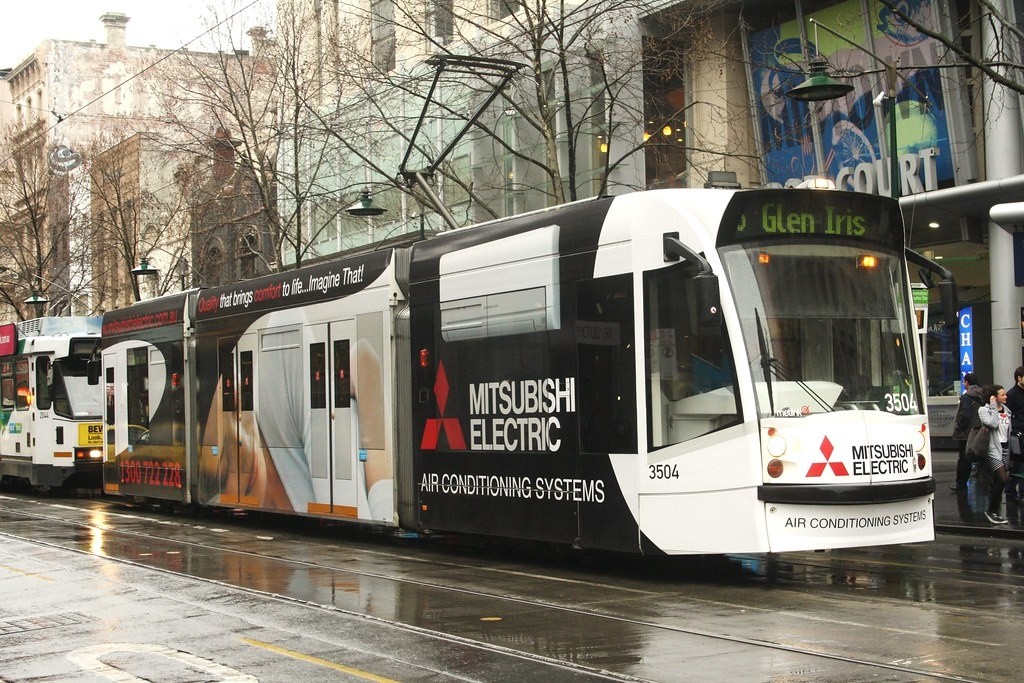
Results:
[971,472,980,479]
[985,510,1008,524]
[949,479,968,492]
[1006,492,1021,502]
[1019,492,1024,503]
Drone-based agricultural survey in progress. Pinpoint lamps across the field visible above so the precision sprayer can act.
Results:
[758,250,770,264]
[863,255,876,267]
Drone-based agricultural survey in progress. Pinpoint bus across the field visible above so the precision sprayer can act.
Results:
[88,52,957,555]
[0,317,103,486]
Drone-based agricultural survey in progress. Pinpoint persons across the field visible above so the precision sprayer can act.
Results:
[946,366,1024,524]
[195,308,395,521]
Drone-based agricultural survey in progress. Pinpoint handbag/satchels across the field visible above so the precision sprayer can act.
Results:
[965,421,990,460]
[1010,435,1023,454]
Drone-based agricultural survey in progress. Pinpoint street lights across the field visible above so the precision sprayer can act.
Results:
[786,19,932,197]
